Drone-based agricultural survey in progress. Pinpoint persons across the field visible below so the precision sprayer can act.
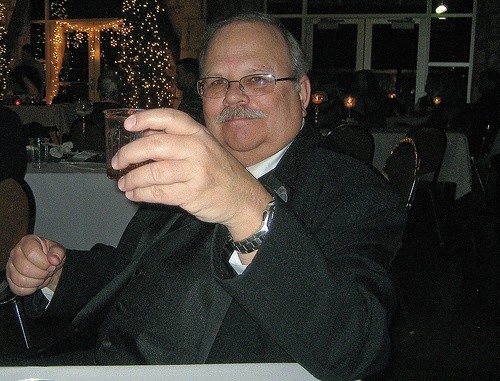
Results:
[0,12,407,381]
[4,43,497,157]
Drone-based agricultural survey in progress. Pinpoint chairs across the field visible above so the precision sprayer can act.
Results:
[0,171,38,353]
[306,69,500,333]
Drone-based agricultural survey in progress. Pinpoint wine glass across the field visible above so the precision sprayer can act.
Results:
[73,98,95,139]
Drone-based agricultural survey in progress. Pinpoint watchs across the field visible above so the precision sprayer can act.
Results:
[223,196,276,253]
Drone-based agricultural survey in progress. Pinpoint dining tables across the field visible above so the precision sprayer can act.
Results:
[0,362,321,381]
[6,103,78,147]
[318,126,473,203]
[385,117,500,157]
[24,160,143,252]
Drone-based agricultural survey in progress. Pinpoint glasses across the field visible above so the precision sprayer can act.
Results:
[196,73,297,98]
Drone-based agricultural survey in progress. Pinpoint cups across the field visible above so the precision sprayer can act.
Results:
[29,138,50,169]
[104,108,151,180]
